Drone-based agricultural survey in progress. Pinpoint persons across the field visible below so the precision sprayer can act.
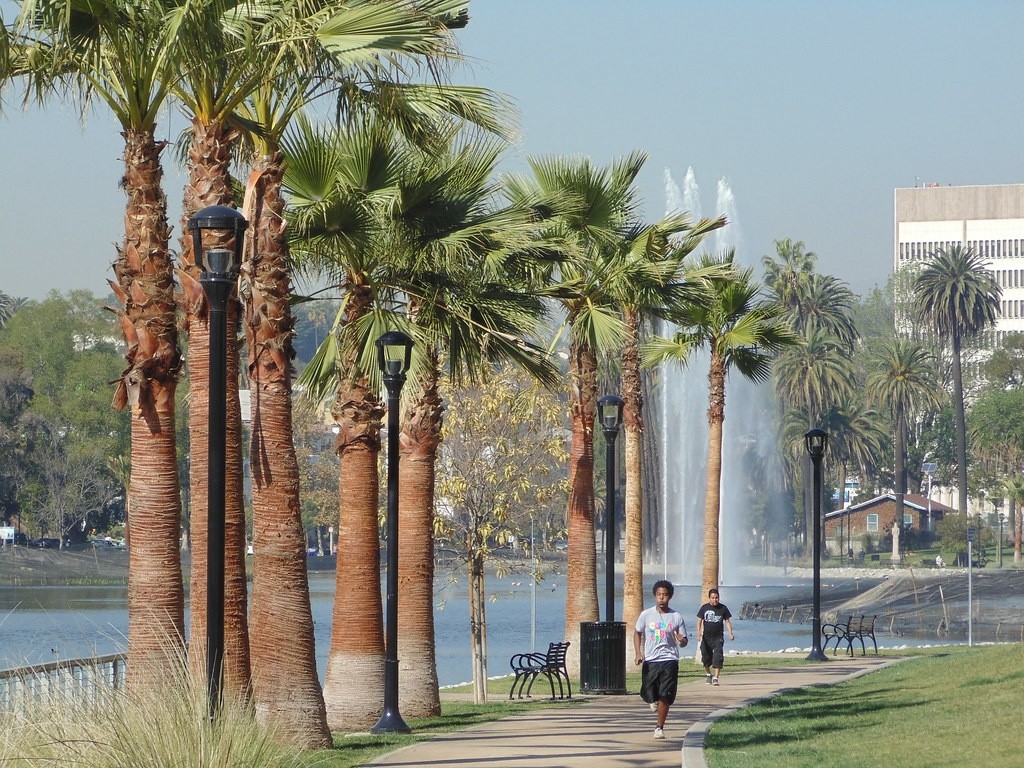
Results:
[697,589,734,687]
[859,548,865,564]
[634,580,688,739]
[846,547,853,566]
[936,555,943,567]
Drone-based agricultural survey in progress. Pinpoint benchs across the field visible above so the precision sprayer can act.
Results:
[822,615,877,658]
[510,642,571,700]
[923,560,938,569]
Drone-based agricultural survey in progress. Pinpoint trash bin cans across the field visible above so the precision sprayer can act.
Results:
[957,552,968,567]
[579,621,627,696]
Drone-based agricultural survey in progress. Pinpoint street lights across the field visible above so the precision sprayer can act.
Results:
[596,395,626,624]
[186,203,250,731]
[803,428,830,661]
[369,331,416,737]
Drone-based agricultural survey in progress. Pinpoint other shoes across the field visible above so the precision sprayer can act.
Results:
[711,678,719,686]
[706,674,712,684]
[650,701,657,713]
[654,726,665,740]
